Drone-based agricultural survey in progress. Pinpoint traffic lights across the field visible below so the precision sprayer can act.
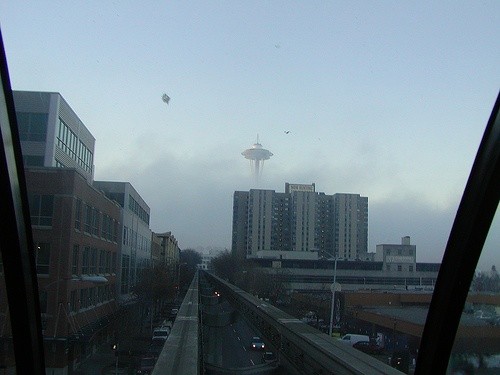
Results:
[111,341,118,350]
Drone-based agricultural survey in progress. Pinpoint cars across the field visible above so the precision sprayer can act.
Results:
[250,336,265,351]
[263,352,274,363]
[130,300,182,375]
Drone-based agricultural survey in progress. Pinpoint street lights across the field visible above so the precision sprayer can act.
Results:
[310,247,338,338]
[177,263,188,290]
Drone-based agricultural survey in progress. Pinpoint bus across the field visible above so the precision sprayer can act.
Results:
[341,317,395,354]
